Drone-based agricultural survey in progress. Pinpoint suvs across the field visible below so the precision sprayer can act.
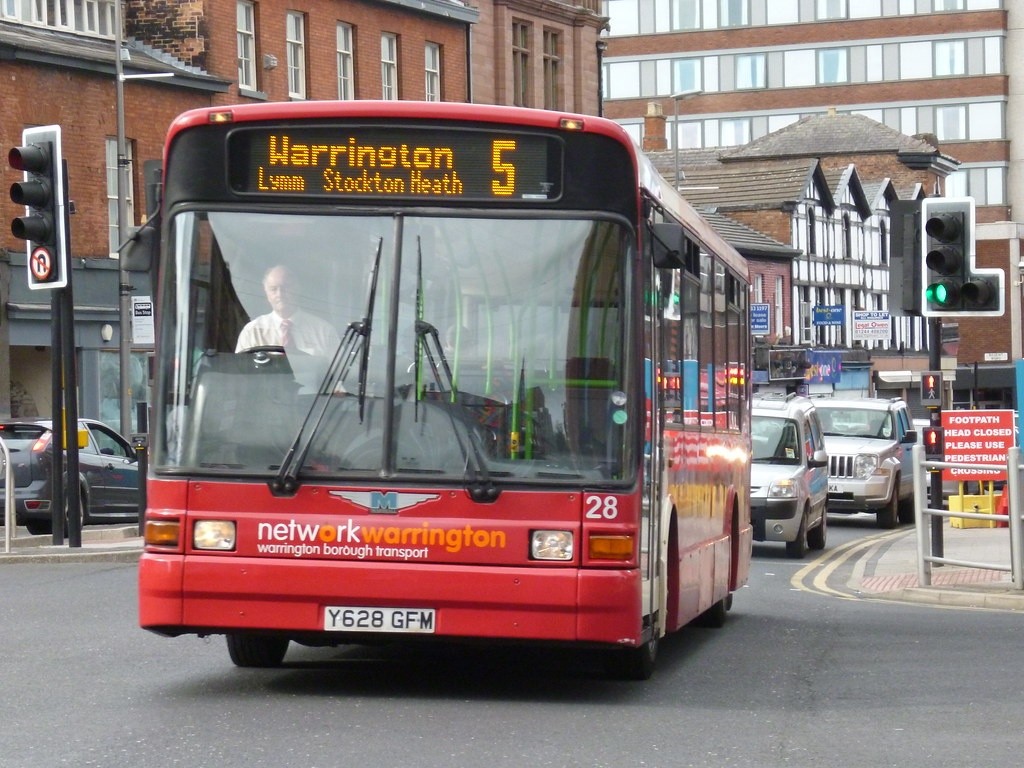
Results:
[738,392,834,562]
[804,394,916,530]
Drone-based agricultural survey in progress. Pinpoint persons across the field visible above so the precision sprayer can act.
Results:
[234,264,352,395]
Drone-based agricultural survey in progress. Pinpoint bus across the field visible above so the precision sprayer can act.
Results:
[98,98,762,689]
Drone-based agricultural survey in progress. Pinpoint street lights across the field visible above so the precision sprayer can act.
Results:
[670,87,705,189]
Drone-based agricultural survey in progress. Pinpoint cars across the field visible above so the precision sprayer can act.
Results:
[911,418,986,504]
[0,418,149,537]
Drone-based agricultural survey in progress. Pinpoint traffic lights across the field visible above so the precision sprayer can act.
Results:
[921,196,976,318]
[964,268,1005,318]
[921,428,945,459]
[921,370,944,408]
[8,139,54,250]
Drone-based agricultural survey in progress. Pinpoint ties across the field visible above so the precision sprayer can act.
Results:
[280,320,295,349]
